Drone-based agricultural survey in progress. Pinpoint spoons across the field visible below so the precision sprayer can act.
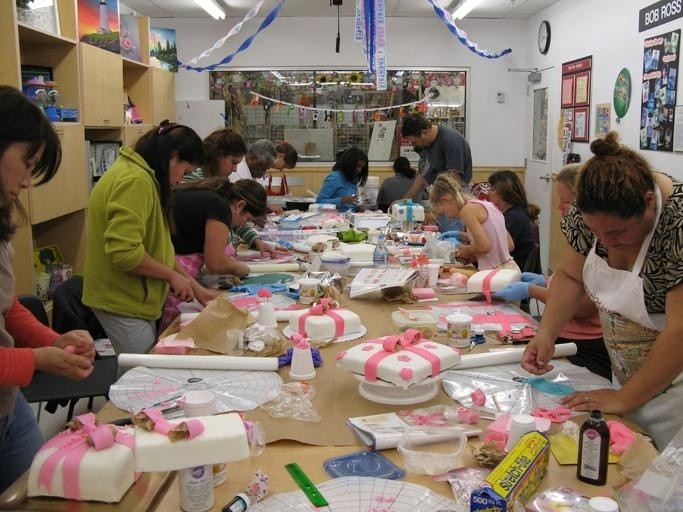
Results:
[397,306,421,321]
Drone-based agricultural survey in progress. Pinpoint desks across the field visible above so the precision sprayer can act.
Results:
[0,211,660,512]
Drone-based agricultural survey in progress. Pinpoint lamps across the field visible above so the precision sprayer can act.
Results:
[193,0,227,20]
[450,0,482,21]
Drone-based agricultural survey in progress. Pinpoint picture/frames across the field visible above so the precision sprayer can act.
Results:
[560,71,591,142]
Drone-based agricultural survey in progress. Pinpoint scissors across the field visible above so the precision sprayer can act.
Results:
[468,337,485,353]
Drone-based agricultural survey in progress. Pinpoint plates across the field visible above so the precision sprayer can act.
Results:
[404,242,424,246]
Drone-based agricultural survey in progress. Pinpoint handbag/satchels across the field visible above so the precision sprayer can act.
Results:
[265,175,288,196]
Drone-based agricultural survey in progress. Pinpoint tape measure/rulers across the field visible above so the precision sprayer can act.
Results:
[285,463,329,508]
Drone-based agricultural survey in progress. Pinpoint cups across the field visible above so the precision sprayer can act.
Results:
[426,263,440,287]
[225,329,245,356]
[35,271,51,306]
[504,414,536,457]
[297,277,320,306]
[445,313,473,349]
[257,303,278,328]
[403,231,424,244]
[180,388,216,419]
[416,265,428,283]
[422,226,439,242]
[288,342,317,381]
[366,230,379,245]
[584,496,620,512]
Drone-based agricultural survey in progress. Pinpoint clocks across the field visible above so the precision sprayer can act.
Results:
[537,21,550,54]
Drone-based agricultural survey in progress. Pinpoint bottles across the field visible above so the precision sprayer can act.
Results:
[443,238,456,264]
[221,492,253,512]
[373,234,388,269]
[576,409,611,485]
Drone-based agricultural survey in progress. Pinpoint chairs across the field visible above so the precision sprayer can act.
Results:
[51,275,123,411]
[15,294,117,426]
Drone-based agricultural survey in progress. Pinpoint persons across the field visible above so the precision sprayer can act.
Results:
[317,113,472,214]
[430,132,682,454]
[1,85,299,492]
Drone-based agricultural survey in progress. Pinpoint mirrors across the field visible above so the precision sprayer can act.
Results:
[205,65,471,167]
